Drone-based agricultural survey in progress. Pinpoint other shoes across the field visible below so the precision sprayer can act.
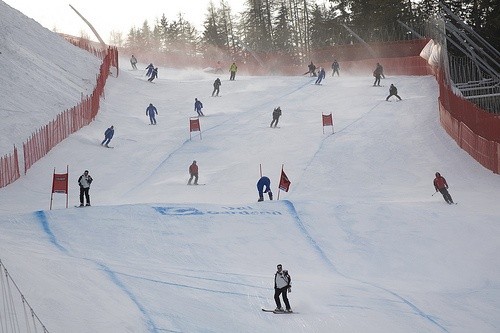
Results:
[274,307,284,311]
[285,309,293,312]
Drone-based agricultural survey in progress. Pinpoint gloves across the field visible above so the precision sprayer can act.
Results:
[288,288,291,293]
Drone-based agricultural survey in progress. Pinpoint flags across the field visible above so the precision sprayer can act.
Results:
[278,170,291,192]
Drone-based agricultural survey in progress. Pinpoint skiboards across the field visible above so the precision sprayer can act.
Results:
[74,204,89,208]
[262,308,299,314]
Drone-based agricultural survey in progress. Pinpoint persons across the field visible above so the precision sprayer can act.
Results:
[433,172,453,203]
[230,63,238,80]
[78,170,93,206]
[146,103,158,125]
[386,83,402,101]
[315,68,326,84]
[332,60,340,76]
[188,161,199,184]
[148,68,158,81]
[102,125,115,147]
[146,64,154,76]
[213,78,221,96]
[373,63,385,85]
[271,106,281,127]
[257,176,273,201]
[194,97,204,115]
[305,62,317,76]
[130,55,137,69]
[275,264,293,312]
